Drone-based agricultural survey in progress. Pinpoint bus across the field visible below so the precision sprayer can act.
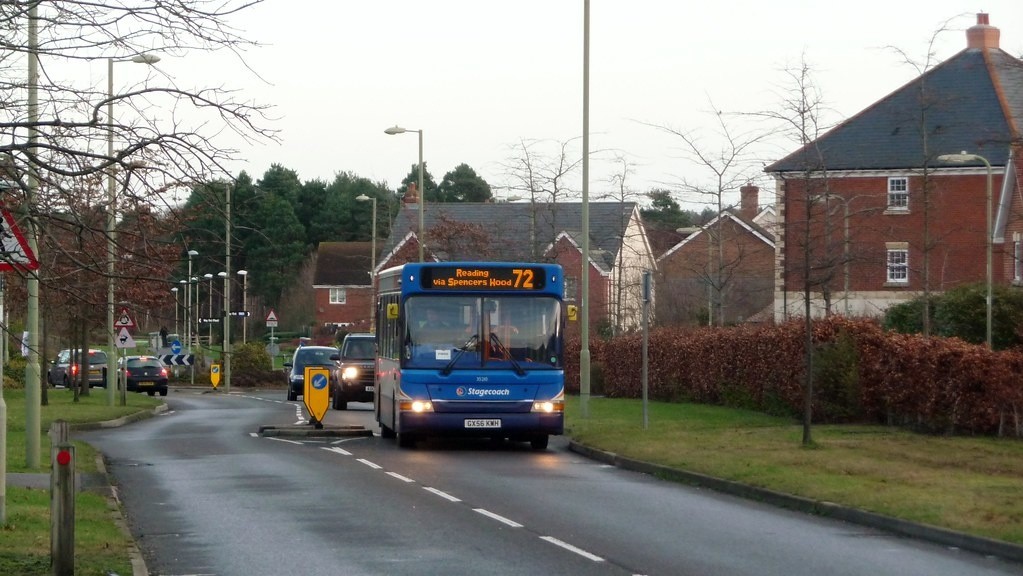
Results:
[373,261,579,450]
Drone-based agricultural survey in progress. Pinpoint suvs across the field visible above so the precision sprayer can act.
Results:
[47,349,108,388]
[330,331,376,409]
[282,341,342,401]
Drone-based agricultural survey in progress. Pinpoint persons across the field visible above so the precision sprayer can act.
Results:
[422,303,452,329]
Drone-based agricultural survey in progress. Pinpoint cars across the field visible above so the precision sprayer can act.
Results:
[116,356,169,397]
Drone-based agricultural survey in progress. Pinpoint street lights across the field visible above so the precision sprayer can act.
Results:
[813,193,850,318]
[936,152,993,350]
[170,249,249,386]
[588,249,616,330]
[105,54,161,405]
[677,226,712,326]
[355,194,379,333]
[384,124,424,261]
